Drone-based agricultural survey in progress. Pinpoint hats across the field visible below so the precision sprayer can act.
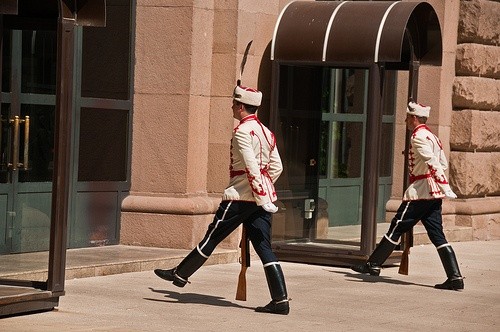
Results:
[406,101,431,118]
[233,85,262,106]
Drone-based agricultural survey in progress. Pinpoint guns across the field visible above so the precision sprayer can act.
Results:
[398,227,413,275]
[235,223,250,301]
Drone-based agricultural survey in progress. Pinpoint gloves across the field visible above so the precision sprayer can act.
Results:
[437,182,457,199]
[261,201,278,213]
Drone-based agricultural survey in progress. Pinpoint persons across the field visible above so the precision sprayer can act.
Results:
[351,99,464,289]
[154,85,290,315]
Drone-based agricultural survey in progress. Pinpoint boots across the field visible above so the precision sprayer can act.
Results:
[154,244,210,287]
[434,243,464,290]
[255,261,289,315]
[350,234,399,277]
